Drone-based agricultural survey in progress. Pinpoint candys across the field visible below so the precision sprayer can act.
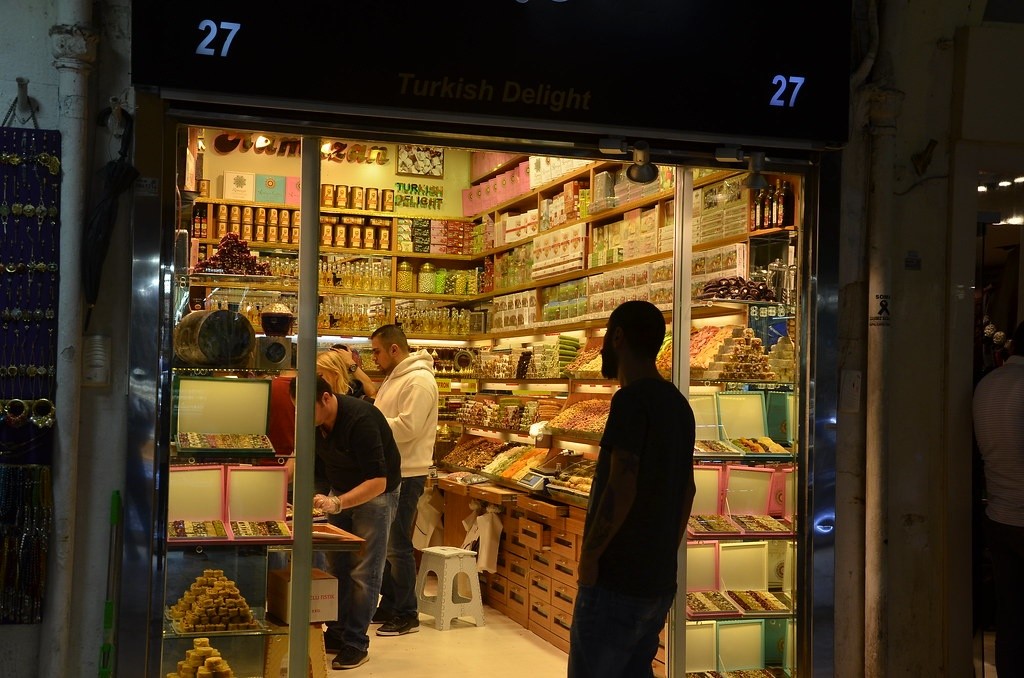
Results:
[397,270,477,295]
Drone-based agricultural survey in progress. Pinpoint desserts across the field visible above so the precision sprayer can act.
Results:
[454,335,609,434]
[193,233,272,276]
[703,326,796,382]
[699,276,776,301]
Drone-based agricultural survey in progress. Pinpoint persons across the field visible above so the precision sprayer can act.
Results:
[316,351,374,404]
[972,321,1024,678]
[330,344,377,396]
[567,300,696,678]
[371,323,439,636]
[285,371,403,671]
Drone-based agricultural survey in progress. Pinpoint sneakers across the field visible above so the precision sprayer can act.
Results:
[375,612,420,636]
[332,645,369,669]
[370,607,393,624]
[324,631,344,653]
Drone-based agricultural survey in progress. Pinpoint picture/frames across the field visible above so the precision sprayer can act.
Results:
[394,143,444,178]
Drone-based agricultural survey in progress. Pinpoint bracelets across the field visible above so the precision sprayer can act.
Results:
[5,398,27,422]
[30,398,56,428]
[331,495,341,512]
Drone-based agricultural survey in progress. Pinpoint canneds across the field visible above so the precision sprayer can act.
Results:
[216,183,394,249]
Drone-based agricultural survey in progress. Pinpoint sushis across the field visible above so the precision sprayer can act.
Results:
[166,638,236,678]
[170,569,257,631]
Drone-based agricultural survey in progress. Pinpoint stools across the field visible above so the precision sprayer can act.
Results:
[415,543,484,631]
[265,616,329,678]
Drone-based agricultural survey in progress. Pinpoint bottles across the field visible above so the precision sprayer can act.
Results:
[773,179,782,228]
[318,259,391,291]
[317,302,390,331]
[764,185,776,228]
[778,180,794,227]
[194,209,200,237]
[198,246,206,262]
[211,295,228,310]
[755,187,767,230]
[750,190,760,232]
[201,210,206,237]
[396,307,471,335]
[259,256,298,277]
[241,296,297,325]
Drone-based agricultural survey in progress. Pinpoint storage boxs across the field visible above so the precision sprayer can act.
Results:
[397,153,748,332]
[267,568,339,626]
[167,375,294,544]
[223,171,301,204]
[686,390,796,678]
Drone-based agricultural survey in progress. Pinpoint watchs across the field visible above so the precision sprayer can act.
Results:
[349,364,358,372]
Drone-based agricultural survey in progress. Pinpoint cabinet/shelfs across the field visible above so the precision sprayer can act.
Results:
[187,198,473,477]
[439,153,801,678]
[685,297,799,678]
[162,271,298,637]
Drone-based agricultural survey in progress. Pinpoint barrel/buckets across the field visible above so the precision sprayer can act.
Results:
[174,310,255,365]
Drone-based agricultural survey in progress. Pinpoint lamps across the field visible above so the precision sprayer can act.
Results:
[619,141,661,185]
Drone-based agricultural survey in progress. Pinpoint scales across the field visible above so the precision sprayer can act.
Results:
[517,467,562,491]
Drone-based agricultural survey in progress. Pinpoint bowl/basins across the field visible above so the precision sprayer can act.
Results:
[261,313,294,337]
